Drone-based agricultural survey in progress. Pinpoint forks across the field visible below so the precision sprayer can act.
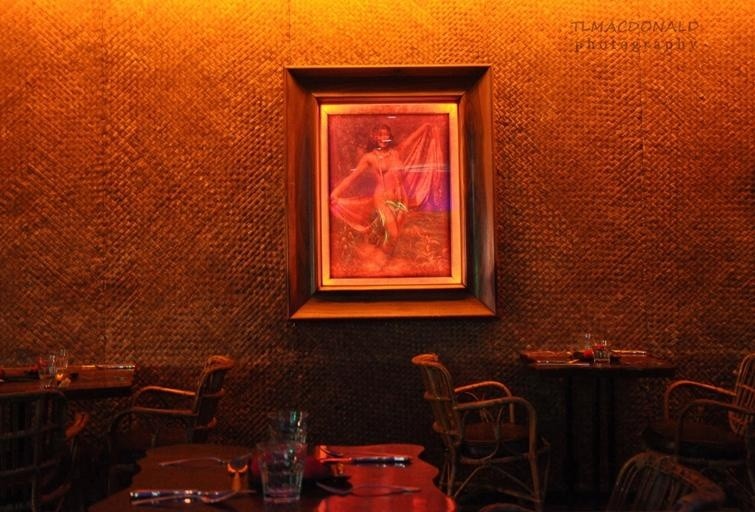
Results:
[316,479,423,496]
[128,491,238,507]
[319,447,412,462]
[158,451,250,468]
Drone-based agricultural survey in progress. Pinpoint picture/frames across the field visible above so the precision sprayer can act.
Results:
[276,63,498,324]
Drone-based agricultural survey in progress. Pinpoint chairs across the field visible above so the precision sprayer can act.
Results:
[604,452,725,512]
[409,351,546,511]
[91,354,235,500]
[661,353,755,504]
[0,392,74,510]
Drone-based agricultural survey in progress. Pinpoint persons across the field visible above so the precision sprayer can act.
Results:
[332,123,438,256]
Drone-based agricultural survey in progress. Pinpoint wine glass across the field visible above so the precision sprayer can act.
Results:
[37,346,70,389]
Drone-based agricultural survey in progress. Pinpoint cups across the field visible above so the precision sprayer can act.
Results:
[580,322,619,369]
[252,409,312,507]
